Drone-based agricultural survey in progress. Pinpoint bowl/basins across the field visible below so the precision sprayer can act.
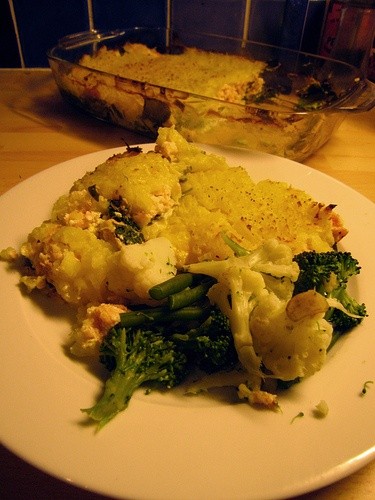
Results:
[49,24,375,163]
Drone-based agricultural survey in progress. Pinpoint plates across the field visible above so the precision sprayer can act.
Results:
[0,143,374,495]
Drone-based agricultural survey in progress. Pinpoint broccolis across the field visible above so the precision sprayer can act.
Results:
[2,124,367,424]
